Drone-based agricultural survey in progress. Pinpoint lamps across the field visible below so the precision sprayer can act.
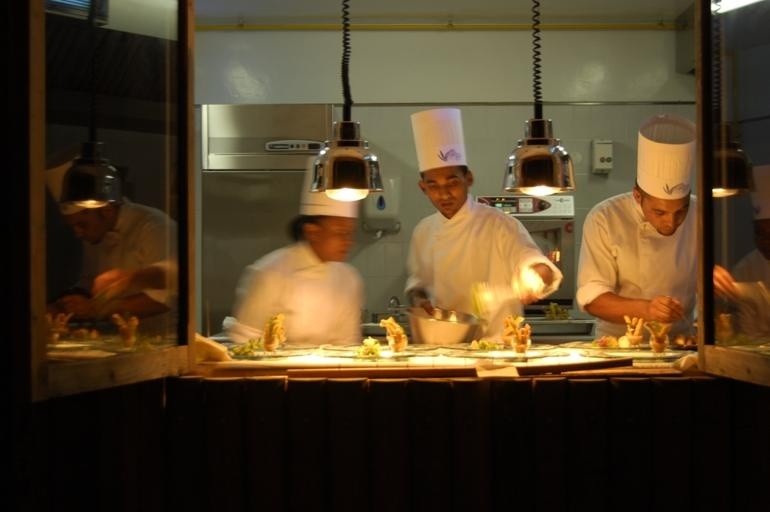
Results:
[502,0,577,197]
[308,0,384,202]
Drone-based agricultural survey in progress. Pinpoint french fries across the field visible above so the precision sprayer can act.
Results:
[109,314,138,337]
[622,315,644,338]
[645,320,672,343]
[515,324,532,346]
[40,311,72,331]
[503,316,525,337]
[263,313,285,342]
[380,317,400,339]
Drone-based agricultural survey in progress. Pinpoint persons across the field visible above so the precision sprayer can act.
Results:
[44,155,179,342]
[711,218,770,349]
[401,165,565,348]
[573,180,698,344]
[222,211,368,352]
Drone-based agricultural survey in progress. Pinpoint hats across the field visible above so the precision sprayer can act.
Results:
[750,165,770,220]
[44,155,89,215]
[410,108,468,173]
[298,155,361,219]
[636,112,697,200]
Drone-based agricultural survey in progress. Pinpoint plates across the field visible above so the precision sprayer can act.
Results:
[45,328,116,352]
[221,342,683,366]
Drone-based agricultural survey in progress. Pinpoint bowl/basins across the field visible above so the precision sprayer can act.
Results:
[402,305,488,348]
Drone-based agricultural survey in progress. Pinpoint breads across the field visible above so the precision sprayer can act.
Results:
[673,333,695,346]
